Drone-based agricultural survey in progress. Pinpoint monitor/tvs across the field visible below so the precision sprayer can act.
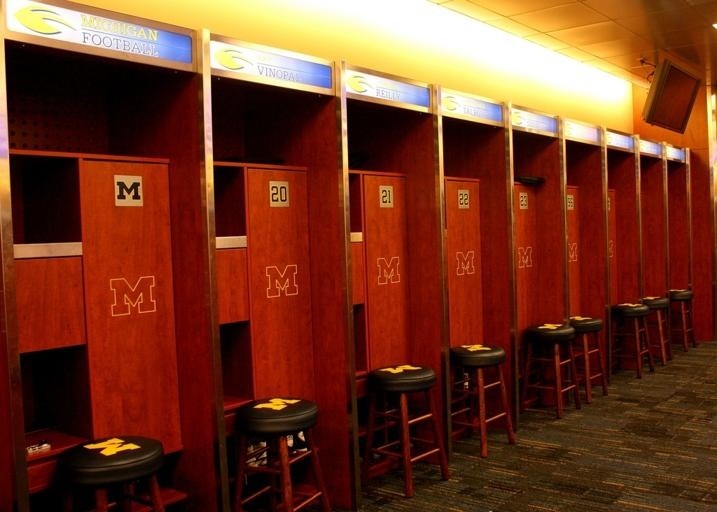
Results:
[642,58,702,134]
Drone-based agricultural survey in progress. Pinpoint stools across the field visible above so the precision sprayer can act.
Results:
[606,303,654,379]
[61,434,165,511]
[569,315,607,403]
[639,295,671,365]
[524,322,581,417]
[666,289,692,352]
[448,344,517,456]
[231,397,333,511]
[360,365,450,497]
